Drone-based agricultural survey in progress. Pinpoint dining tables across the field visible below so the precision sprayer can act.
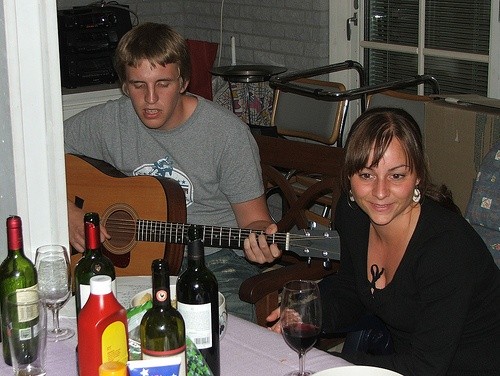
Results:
[0,296,355,376]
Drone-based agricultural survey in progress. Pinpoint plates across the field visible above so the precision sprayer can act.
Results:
[311,366,403,376]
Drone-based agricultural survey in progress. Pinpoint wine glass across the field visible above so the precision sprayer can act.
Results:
[280,280,321,376]
[35,244,75,341]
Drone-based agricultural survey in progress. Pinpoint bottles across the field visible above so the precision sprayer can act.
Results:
[0,216,37,366]
[77,275,130,376]
[176,224,220,376]
[140,258,187,376]
[74,213,117,316]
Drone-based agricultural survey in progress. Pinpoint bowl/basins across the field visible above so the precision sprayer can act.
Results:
[129,285,228,341]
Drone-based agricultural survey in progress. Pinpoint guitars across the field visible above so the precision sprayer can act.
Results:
[66,153,341,276]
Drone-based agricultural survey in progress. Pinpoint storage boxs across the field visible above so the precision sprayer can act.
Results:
[421,96,500,219]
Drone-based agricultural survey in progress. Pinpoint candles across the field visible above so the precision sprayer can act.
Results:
[231,36,237,66]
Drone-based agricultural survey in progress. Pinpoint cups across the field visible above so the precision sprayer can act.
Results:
[6,289,47,376]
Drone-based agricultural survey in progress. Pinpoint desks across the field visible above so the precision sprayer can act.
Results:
[207,61,288,137]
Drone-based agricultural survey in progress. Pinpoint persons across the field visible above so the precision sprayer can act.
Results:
[64,21,281,329]
[266,105,500,376]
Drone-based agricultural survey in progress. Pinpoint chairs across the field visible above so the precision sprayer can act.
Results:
[238,80,500,327]
